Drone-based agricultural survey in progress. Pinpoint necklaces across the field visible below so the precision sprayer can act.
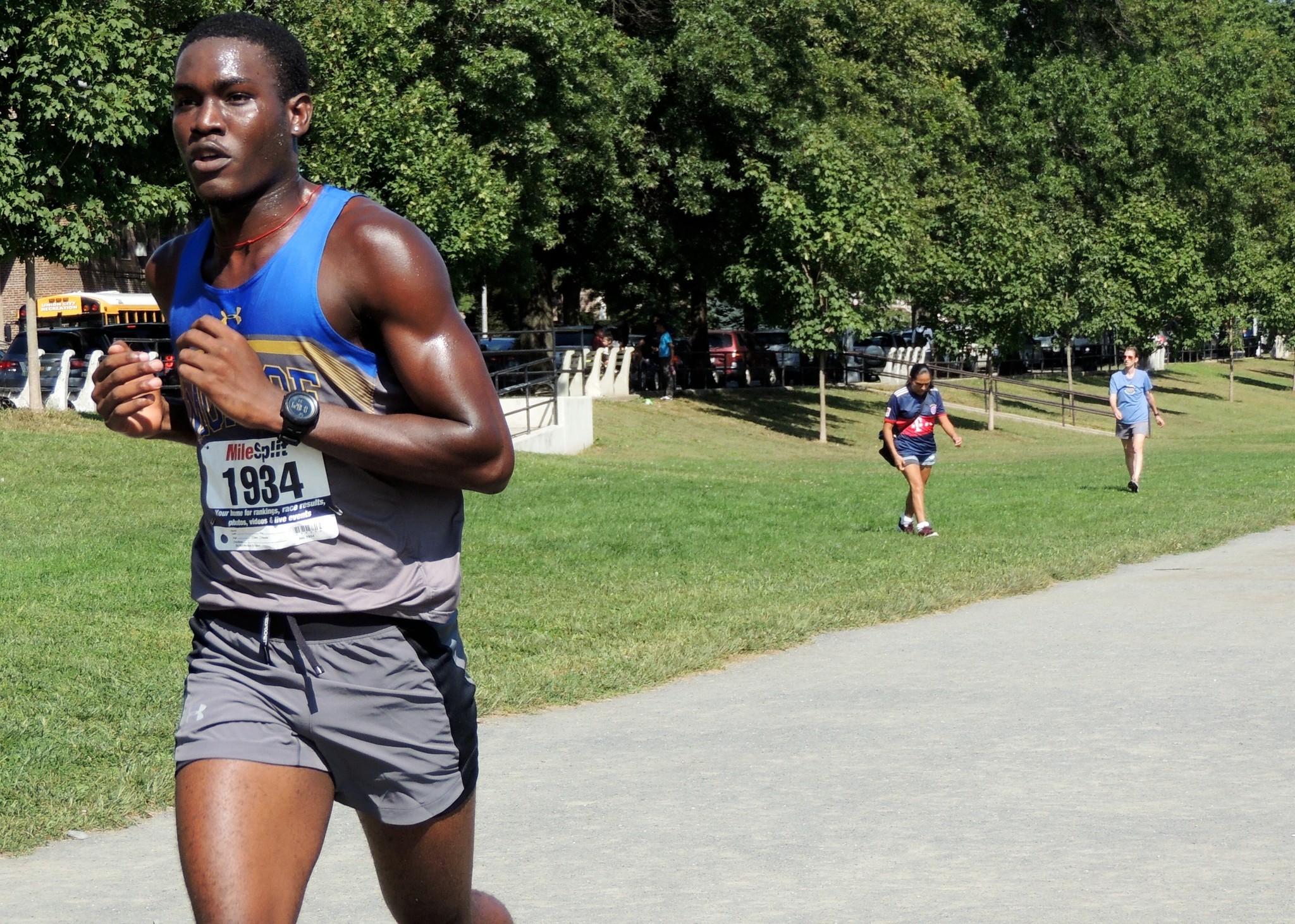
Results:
[213,185,322,249]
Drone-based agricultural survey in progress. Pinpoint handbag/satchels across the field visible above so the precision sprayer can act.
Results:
[876,430,896,468]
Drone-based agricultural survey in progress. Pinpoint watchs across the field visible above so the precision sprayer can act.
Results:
[276,390,319,446]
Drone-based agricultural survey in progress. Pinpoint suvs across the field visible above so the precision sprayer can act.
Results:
[690,329,779,387]
[850,327,990,380]
[752,327,810,384]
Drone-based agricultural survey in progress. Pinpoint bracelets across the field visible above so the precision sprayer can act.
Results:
[1154,413,1159,416]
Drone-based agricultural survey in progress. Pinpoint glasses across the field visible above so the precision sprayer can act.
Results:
[1123,355,1135,359]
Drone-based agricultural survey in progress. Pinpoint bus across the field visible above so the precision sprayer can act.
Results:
[4,290,166,343]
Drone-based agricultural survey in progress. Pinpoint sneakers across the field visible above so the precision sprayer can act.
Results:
[896,517,914,533]
[918,525,939,539]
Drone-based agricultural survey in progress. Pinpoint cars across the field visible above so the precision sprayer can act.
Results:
[0,327,103,415]
[478,316,721,393]
[100,322,181,404]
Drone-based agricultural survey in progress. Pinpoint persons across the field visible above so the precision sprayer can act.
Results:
[853,332,865,347]
[788,340,845,385]
[1108,344,1165,493]
[882,363,962,538]
[652,320,676,401]
[90,10,517,924]
[1217,320,1228,357]
[591,320,661,391]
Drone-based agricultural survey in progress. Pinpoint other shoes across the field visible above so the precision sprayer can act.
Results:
[1128,480,1139,492]
